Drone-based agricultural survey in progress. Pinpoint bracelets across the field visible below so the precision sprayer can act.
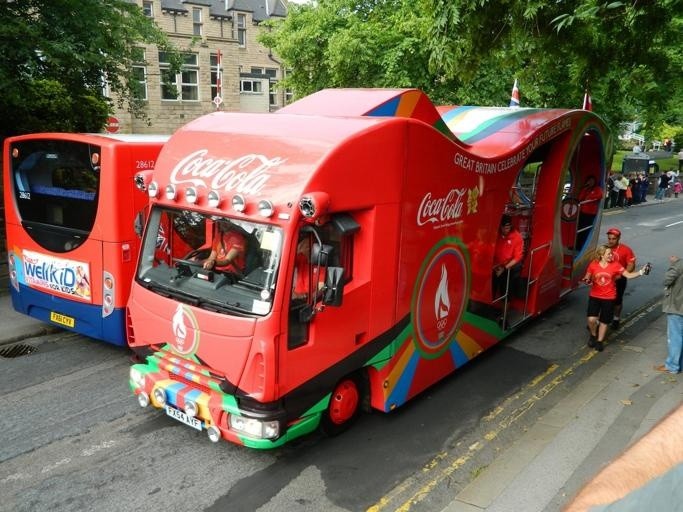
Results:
[639,269,643,276]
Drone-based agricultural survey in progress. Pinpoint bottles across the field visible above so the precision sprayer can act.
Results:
[644,262,650,274]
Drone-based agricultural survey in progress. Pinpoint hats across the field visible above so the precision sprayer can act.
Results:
[604,228,621,237]
[499,214,512,226]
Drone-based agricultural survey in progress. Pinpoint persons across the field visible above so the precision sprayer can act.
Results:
[200,218,246,276]
[603,226,638,331]
[574,175,603,252]
[290,234,327,300]
[492,213,525,300]
[602,167,682,210]
[653,246,683,372]
[580,243,652,352]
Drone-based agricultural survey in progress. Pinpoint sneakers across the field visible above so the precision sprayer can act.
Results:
[587,335,604,352]
[610,319,620,330]
[653,364,679,374]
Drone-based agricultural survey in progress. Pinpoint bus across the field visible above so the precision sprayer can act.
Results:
[2,130,175,351]
[122,87,615,451]
